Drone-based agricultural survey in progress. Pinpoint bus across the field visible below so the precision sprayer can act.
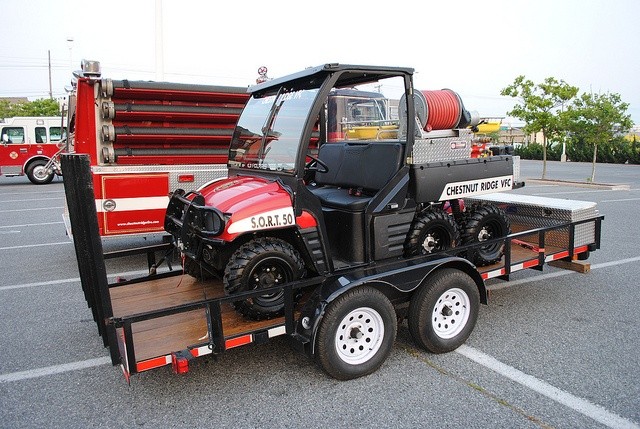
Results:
[0,116,68,184]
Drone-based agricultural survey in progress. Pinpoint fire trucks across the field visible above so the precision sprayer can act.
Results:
[61,59,393,256]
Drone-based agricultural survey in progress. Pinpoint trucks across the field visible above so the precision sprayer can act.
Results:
[58,155,604,387]
[162,62,525,319]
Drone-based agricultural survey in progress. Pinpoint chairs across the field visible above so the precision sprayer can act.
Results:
[306,143,403,265]
